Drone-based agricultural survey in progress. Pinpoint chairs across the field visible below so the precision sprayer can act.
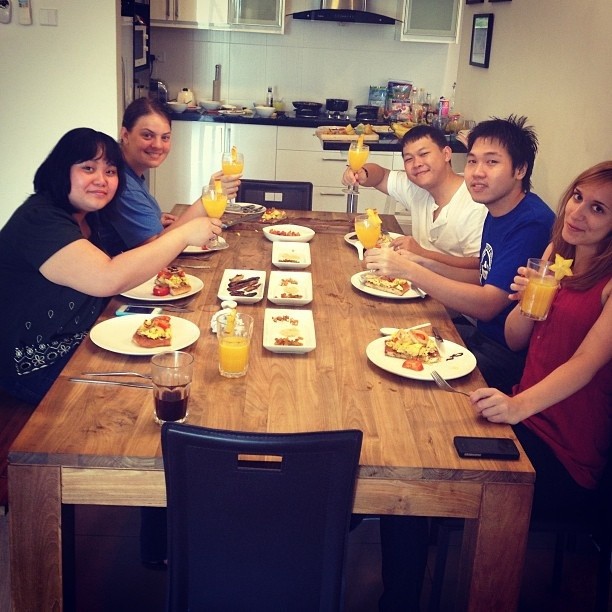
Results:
[235,179,314,209]
[161,422,363,611]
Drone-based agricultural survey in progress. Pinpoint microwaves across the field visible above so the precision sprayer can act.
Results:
[135,24,150,69]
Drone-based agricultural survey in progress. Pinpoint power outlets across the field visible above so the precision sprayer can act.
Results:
[37,7,57,28]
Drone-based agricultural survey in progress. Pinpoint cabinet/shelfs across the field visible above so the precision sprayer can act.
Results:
[394,2,462,44]
[147,123,277,213]
[227,0,287,32]
[276,127,394,214]
[149,0,199,27]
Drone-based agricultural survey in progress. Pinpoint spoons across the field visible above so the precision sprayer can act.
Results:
[128,297,196,308]
[350,240,366,260]
[80,364,167,380]
[380,321,431,336]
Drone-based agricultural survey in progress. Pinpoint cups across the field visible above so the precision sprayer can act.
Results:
[151,350,194,428]
[216,313,254,378]
[520,258,559,322]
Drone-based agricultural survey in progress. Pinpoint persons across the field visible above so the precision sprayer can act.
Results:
[364,121,557,388]
[470,160,612,511]
[344,126,488,269]
[106,96,241,252]
[1,128,224,419]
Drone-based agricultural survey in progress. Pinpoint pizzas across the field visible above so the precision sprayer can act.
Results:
[384,329,438,362]
[153,266,191,295]
[359,273,410,295]
[133,314,173,347]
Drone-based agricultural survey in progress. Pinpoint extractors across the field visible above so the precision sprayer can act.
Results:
[287,0,404,25]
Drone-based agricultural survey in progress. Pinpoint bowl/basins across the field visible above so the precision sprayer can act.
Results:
[255,106,275,118]
[222,105,236,109]
[200,100,220,110]
[167,102,188,113]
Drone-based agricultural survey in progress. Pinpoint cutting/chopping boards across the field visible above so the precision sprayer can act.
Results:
[315,129,379,140]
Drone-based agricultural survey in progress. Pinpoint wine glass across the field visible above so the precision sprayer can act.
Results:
[354,215,383,280]
[201,185,230,252]
[342,143,370,195]
[223,151,244,210]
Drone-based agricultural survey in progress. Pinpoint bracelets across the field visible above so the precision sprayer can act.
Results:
[346,164,369,179]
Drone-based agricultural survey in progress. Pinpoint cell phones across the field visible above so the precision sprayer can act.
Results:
[454,436,520,461]
[115,305,163,317]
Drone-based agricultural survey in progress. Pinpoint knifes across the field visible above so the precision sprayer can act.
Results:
[221,213,261,230]
[68,378,172,390]
[411,282,426,300]
[178,264,211,269]
[432,326,449,360]
[213,64,221,101]
[378,221,397,247]
[160,307,194,313]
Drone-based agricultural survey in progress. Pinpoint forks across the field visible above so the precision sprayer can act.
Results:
[430,370,471,399]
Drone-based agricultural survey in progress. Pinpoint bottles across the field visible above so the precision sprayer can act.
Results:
[426,91,433,124]
[411,87,420,123]
[420,87,426,107]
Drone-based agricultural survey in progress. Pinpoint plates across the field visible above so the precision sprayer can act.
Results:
[217,268,266,305]
[182,236,228,253]
[267,271,314,308]
[351,270,428,299]
[344,231,406,261]
[119,274,204,302]
[262,224,315,243]
[272,241,312,270]
[223,201,267,214]
[262,308,317,355]
[217,111,246,117]
[365,336,478,381]
[90,314,200,356]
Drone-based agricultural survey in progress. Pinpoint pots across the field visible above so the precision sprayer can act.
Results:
[292,101,322,110]
[356,105,379,119]
[326,99,348,112]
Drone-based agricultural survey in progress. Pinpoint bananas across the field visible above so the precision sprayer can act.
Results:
[390,119,414,139]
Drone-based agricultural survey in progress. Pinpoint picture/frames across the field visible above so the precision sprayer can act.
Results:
[468,13,494,68]
